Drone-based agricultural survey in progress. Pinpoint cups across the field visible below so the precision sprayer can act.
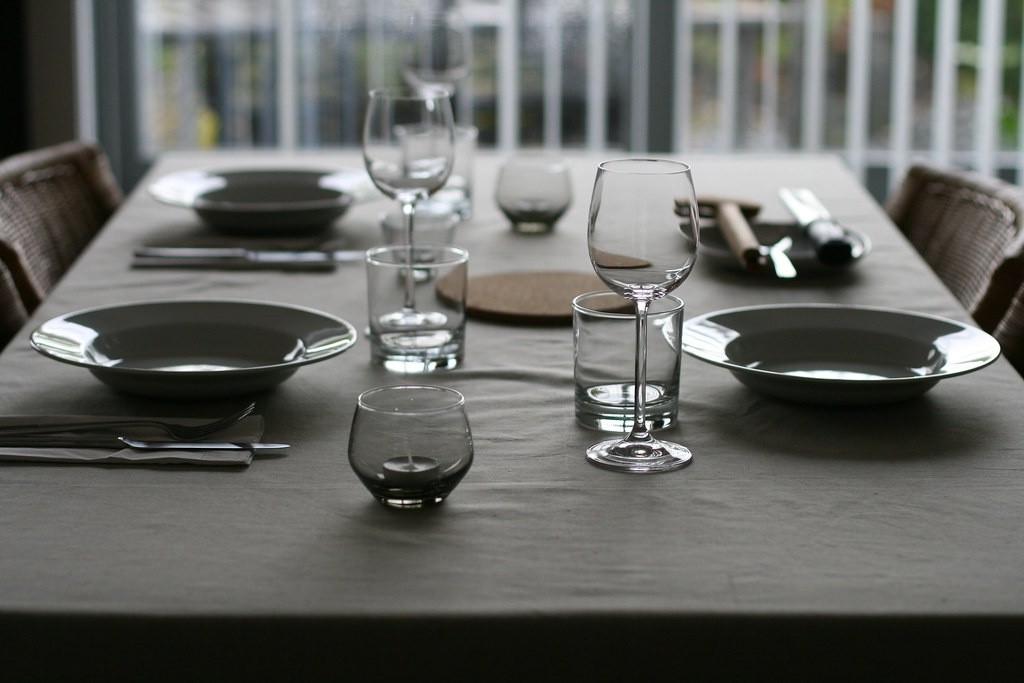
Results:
[364,242,469,374]
[401,123,478,221]
[347,383,475,511]
[573,290,686,435]
[380,212,460,281]
[495,159,573,236]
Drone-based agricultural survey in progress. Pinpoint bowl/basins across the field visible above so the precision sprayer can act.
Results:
[146,164,384,239]
[662,302,1001,409]
[29,297,358,417]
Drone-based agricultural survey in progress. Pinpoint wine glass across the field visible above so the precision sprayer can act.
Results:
[361,85,456,332]
[586,158,700,473]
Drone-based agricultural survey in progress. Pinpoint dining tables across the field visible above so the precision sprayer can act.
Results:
[0,149,1024,683]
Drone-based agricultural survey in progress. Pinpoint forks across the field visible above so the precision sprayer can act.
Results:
[0,401,256,442]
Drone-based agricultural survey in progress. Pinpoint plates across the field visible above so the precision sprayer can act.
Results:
[678,221,872,267]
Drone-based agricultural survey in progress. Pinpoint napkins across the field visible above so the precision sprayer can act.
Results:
[0,412,265,465]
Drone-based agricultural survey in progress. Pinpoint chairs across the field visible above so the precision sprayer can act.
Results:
[881,159,1022,376]
[2,135,123,350]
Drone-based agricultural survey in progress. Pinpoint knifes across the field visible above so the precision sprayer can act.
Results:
[132,244,370,264]
[781,187,853,268]
[0,432,291,452]
